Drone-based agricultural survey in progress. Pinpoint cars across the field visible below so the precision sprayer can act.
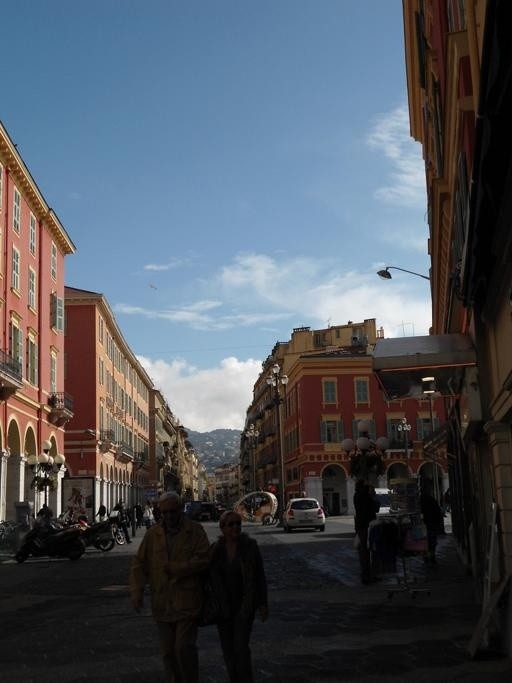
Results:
[217,507,226,517]
[283,498,326,533]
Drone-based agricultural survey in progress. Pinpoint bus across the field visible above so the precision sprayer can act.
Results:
[198,502,215,520]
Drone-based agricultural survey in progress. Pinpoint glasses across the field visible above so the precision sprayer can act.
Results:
[223,520,243,528]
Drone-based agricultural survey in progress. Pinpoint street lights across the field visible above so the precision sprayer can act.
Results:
[28,440,65,506]
[265,363,288,523]
[245,424,259,493]
[397,418,412,451]
[338,420,389,508]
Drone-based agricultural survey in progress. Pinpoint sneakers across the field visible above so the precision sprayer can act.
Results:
[357,576,376,588]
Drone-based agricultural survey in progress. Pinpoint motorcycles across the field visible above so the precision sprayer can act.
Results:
[14,510,124,564]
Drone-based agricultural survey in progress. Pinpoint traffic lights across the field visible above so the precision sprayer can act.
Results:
[266,483,278,493]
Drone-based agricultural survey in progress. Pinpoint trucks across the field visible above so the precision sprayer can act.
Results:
[185,500,202,514]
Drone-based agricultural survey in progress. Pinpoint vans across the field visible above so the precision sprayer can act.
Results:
[374,487,396,514]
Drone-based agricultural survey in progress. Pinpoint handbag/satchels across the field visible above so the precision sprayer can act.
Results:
[197,590,222,624]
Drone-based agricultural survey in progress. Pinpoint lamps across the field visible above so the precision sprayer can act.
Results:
[376,266,430,279]
[84,428,95,437]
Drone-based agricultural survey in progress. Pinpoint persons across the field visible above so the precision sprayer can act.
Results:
[199,508,270,680]
[133,500,143,530]
[354,477,382,569]
[130,488,212,681]
[95,500,107,522]
[152,503,161,521]
[113,498,132,543]
[421,477,444,569]
[142,497,155,529]
[37,503,54,520]
[444,487,452,511]
